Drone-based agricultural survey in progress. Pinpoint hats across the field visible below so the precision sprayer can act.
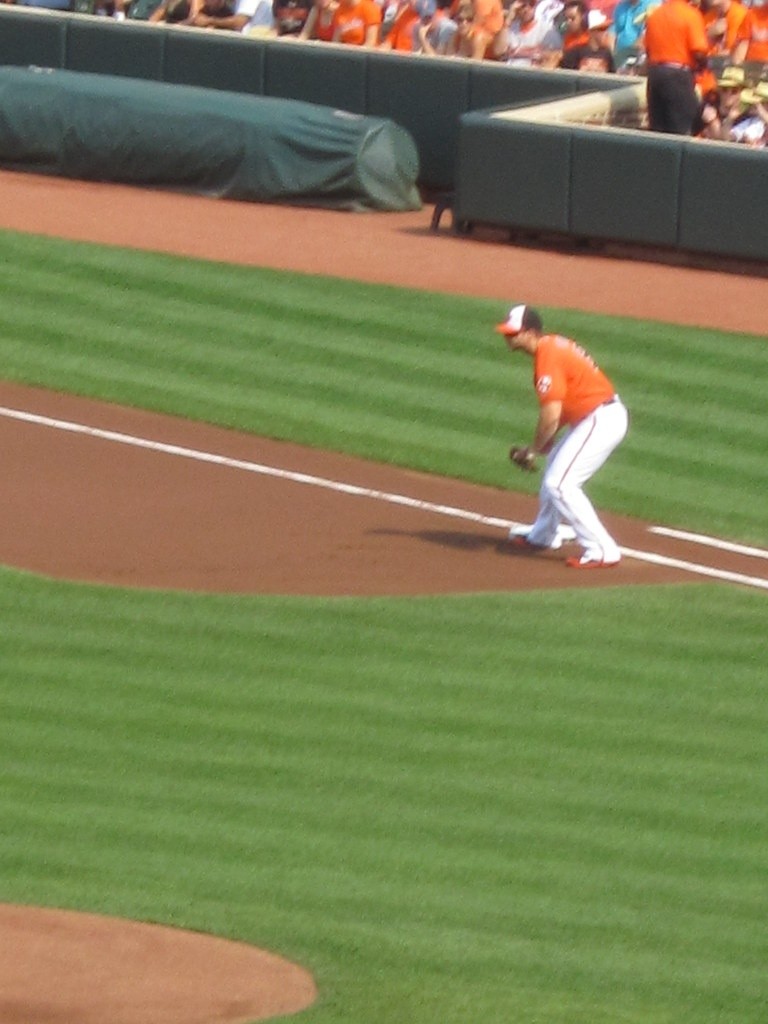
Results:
[495,303,542,334]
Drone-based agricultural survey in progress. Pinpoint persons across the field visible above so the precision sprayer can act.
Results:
[496,305,628,569]
[6,1,768,156]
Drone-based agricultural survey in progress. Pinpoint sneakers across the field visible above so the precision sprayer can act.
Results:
[563,554,621,567]
[511,534,549,551]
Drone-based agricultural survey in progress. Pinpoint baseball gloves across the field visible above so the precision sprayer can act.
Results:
[508,445,539,472]
